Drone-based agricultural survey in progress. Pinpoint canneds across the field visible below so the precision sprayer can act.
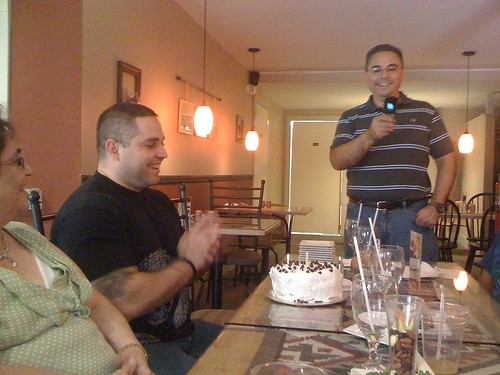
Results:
[195,210,202,221]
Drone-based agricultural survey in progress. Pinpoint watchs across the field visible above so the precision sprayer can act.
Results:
[430,200,446,212]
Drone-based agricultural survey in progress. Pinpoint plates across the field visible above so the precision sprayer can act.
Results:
[402,260,438,279]
[298,239,335,266]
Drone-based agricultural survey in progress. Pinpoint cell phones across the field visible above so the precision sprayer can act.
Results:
[384,96,397,113]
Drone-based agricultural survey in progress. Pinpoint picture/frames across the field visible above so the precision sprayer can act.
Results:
[178,99,195,135]
[117,60,141,104]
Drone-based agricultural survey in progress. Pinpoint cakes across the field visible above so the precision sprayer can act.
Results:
[269,260,344,303]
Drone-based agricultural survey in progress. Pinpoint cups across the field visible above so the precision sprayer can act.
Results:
[249,360,329,375]
[345,218,470,375]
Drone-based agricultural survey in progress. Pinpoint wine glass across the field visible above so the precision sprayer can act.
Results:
[349,274,399,375]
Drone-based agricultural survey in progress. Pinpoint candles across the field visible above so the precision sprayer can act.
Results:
[306,249,309,266]
[287,252,290,266]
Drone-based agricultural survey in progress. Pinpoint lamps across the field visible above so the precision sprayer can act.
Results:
[194,0,213,138]
[458,49,476,154]
[245,48,260,150]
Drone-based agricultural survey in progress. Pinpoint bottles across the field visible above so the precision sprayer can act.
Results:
[468,199,476,215]
[459,195,469,214]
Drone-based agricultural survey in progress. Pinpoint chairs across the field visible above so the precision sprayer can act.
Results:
[434,192,496,277]
[206,177,266,303]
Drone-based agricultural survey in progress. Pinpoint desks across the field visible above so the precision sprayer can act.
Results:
[216,205,313,274]
[185,324,500,375]
[182,215,281,309]
[439,211,496,244]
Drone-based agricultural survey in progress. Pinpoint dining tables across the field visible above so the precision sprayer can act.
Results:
[225,254,500,345]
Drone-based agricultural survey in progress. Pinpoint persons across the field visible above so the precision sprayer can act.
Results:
[0,119,157,375]
[329,44,459,262]
[50,103,222,375]
[471,231,500,305]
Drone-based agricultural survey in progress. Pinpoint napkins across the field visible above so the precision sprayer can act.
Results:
[403,261,437,279]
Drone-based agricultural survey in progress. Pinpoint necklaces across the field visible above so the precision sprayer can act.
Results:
[0,230,17,268]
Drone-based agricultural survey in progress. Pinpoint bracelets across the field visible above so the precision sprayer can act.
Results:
[119,343,148,359]
[181,258,198,280]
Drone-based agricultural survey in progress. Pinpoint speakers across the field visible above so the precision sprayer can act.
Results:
[250,72,259,86]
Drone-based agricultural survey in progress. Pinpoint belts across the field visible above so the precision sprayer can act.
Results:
[349,198,423,209]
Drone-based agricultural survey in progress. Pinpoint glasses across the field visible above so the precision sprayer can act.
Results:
[0,155,29,171]
[366,66,402,74]
